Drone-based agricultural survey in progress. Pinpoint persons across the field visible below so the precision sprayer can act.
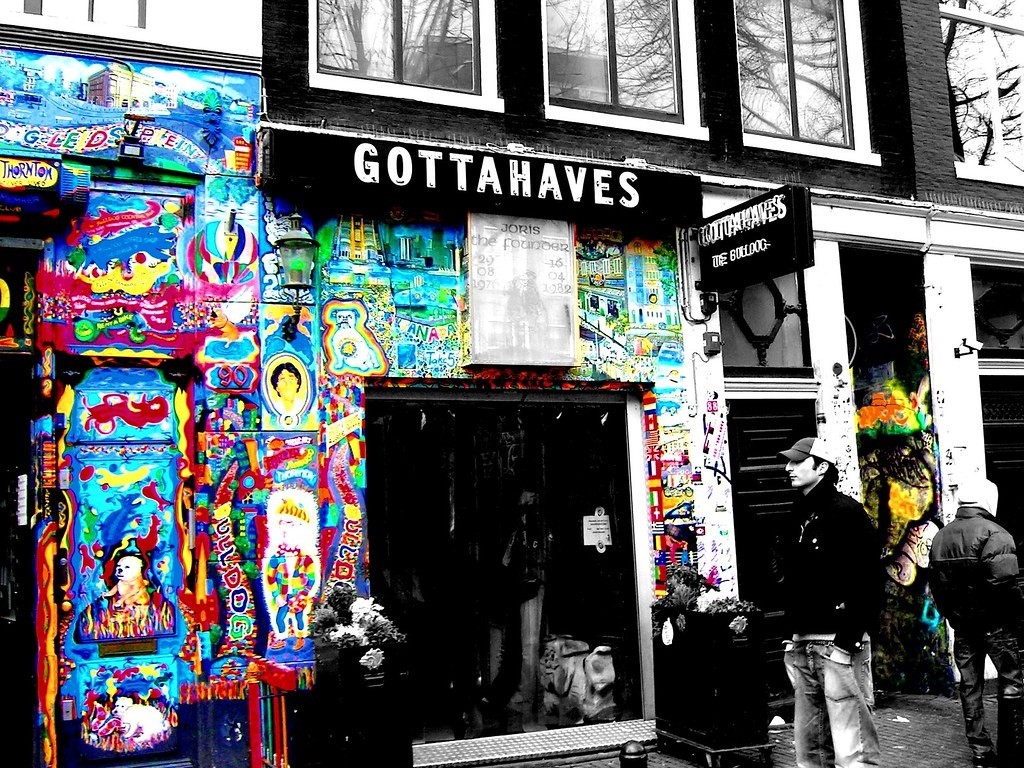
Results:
[770,437,882,768]
[928,476,1024,768]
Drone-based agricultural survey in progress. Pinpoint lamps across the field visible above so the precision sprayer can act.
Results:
[274,206,321,342]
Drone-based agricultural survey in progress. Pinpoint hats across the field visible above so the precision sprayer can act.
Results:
[777,437,836,465]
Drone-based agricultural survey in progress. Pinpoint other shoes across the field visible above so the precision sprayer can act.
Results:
[972,752,996,767]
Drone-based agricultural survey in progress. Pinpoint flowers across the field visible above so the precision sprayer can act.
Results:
[308,585,408,671]
[655,563,757,615]
